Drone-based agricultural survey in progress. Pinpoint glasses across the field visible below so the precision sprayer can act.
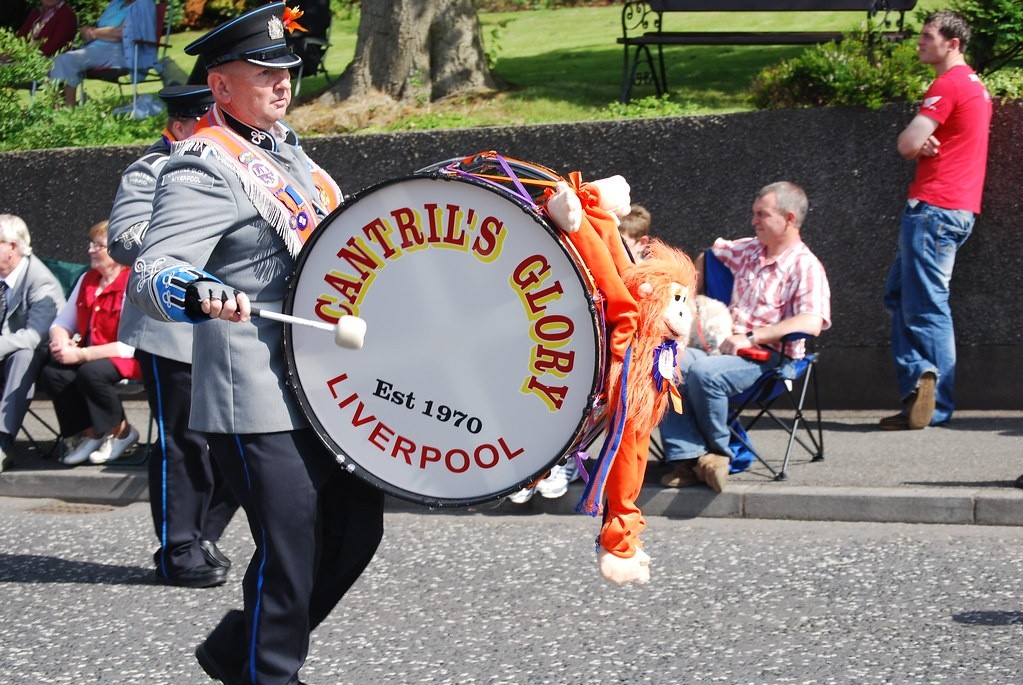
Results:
[89,240,107,251]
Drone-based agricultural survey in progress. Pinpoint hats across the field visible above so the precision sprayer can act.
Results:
[184,1,303,70]
[156,84,216,123]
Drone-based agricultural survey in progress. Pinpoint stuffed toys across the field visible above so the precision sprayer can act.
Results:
[544,174,698,586]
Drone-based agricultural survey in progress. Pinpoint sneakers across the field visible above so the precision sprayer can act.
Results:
[661,460,703,487]
[691,453,731,493]
[58,431,115,465]
[89,424,139,464]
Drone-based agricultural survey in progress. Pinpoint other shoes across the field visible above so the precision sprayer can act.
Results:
[906,372,936,430]
[879,412,908,430]
[0,446,16,475]
[199,539,231,571]
[195,641,309,684]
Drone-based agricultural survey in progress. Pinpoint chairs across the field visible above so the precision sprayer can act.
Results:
[79,0,174,110]
[704,249,823,482]
[19,259,155,466]
[284,0,333,97]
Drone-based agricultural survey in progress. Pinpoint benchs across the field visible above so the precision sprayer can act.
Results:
[616,0,917,104]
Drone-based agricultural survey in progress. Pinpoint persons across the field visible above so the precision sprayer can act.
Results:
[0,0,159,110]
[659,181,832,492]
[40,220,144,466]
[0,214,67,474]
[506,203,650,504]
[881,14,994,431]
[108,0,383,685]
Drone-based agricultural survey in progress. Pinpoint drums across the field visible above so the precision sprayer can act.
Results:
[279,153,640,510]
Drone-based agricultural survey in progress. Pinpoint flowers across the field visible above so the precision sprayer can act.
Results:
[282,6,308,34]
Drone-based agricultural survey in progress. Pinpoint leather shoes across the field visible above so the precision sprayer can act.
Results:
[154,564,227,588]
